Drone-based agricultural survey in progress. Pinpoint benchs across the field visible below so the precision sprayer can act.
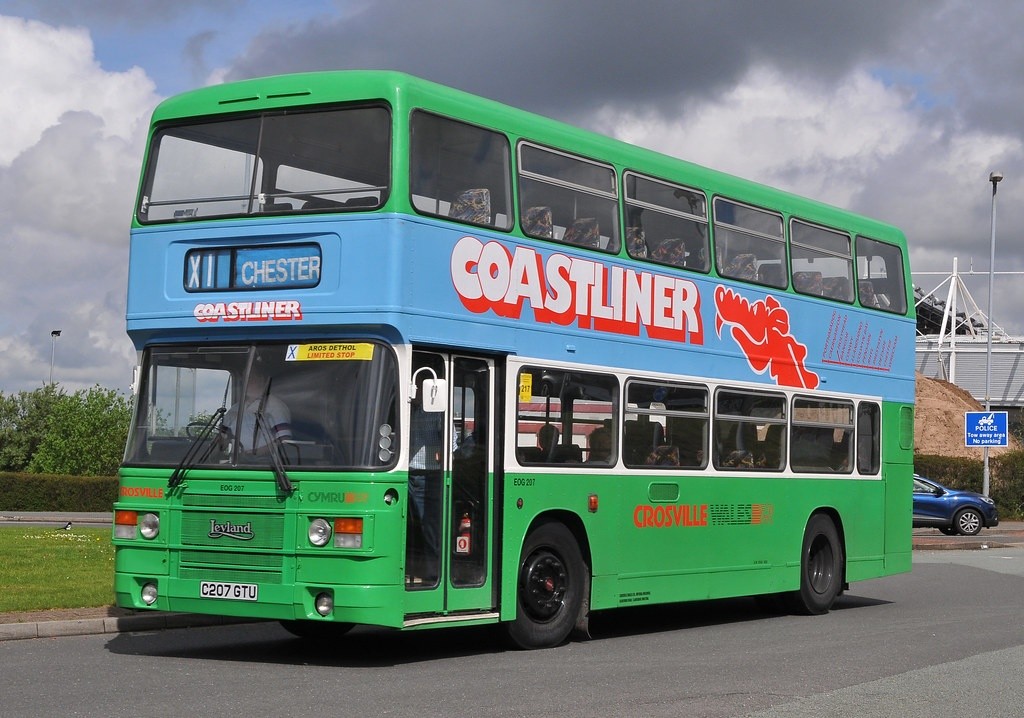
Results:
[763,424,834,470]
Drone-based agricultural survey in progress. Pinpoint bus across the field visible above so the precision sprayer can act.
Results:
[111,70,914,650]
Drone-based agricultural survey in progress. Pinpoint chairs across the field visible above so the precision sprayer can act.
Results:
[521,206,553,239]
[517,425,583,464]
[696,448,721,467]
[858,282,880,307]
[562,218,600,249]
[263,197,379,212]
[757,264,786,287]
[650,238,686,268]
[447,189,491,225]
[631,445,681,467]
[588,428,611,460]
[792,272,823,296]
[823,277,850,302]
[725,253,759,282]
[694,247,724,275]
[624,420,664,451]
[728,424,758,448]
[606,226,647,259]
[722,450,754,468]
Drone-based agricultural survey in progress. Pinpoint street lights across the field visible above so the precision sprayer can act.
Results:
[49,330,61,388]
[981,171,1004,497]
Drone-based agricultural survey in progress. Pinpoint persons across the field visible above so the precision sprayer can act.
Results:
[407,374,458,581]
[533,425,578,463]
[586,428,612,464]
[216,362,292,463]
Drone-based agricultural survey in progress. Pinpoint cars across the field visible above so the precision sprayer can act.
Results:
[912,474,999,535]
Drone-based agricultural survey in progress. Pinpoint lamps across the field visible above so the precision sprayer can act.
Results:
[915,286,985,328]
